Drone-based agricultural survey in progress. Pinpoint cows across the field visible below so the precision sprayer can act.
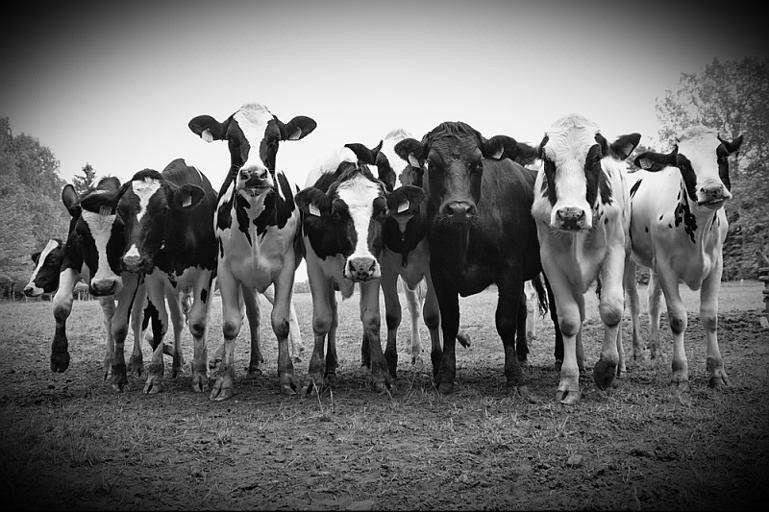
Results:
[23,101,744,407]
